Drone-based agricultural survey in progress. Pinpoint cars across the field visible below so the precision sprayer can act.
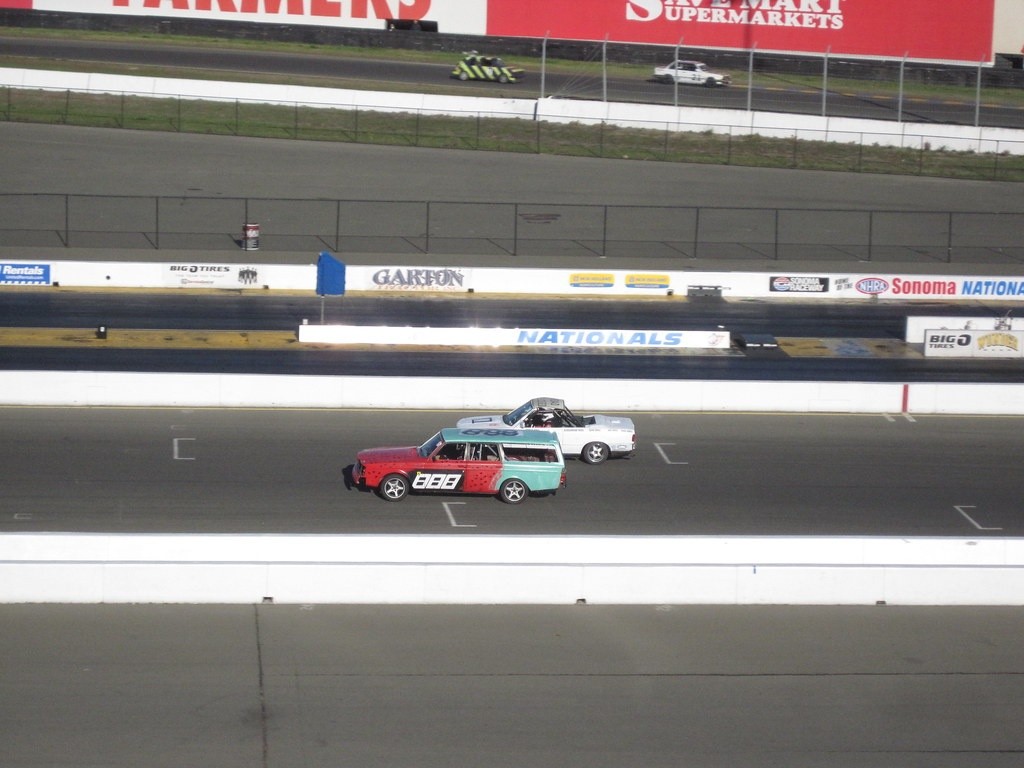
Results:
[353,429,567,505]
[451,54,524,83]
[654,60,732,89]
[455,397,636,465]
